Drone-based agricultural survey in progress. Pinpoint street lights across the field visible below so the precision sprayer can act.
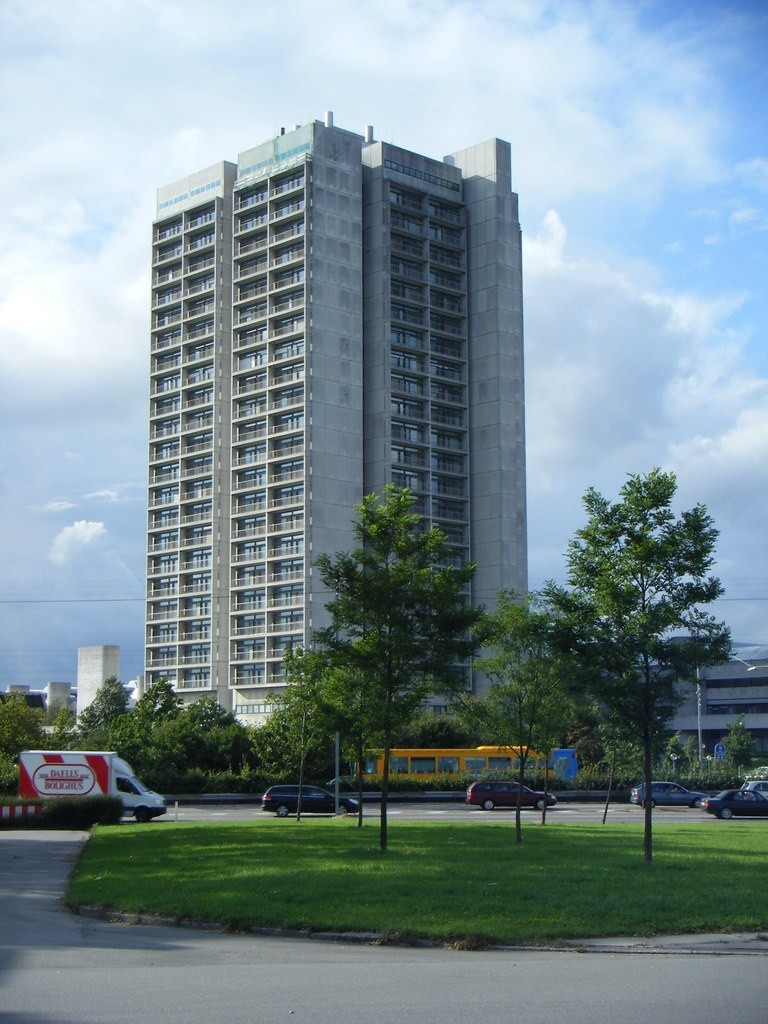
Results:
[670,753,678,782]
[706,754,713,778]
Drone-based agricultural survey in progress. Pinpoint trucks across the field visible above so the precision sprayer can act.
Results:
[13,747,168,824]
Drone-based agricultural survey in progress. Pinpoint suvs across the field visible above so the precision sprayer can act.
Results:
[739,780,768,801]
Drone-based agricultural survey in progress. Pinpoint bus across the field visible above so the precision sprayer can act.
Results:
[352,744,580,791]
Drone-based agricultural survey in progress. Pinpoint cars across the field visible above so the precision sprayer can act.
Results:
[629,780,709,809]
[465,779,558,811]
[700,788,768,819]
[325,775,351,785]
[261,784,359,818]
